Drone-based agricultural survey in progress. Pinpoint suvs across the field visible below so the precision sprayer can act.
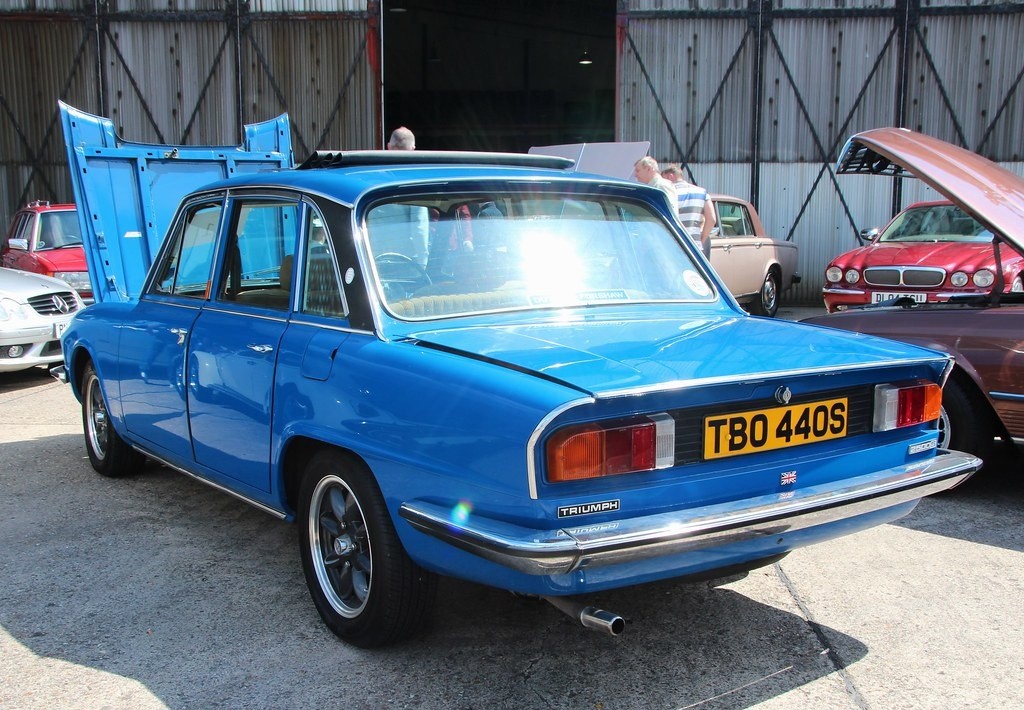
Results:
[0,200,95,306]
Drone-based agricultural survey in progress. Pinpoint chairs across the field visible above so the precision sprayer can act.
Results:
[279,255,342,314]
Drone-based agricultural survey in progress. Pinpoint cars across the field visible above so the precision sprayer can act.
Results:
[51,98,1024,650]
[0,267,88,372]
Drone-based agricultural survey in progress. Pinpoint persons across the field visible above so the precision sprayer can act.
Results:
[429,200,508,279]
[634,156,716,252]
[366,127,429,278]
